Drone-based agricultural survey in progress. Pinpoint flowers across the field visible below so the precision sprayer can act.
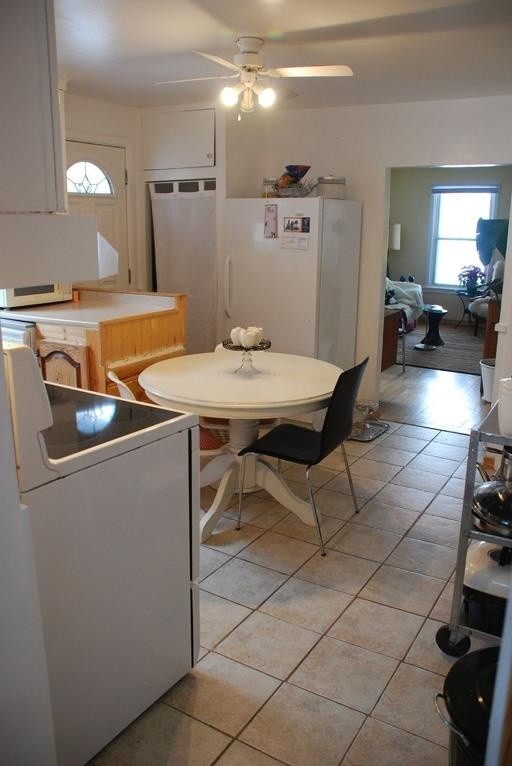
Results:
[457,264,487,289]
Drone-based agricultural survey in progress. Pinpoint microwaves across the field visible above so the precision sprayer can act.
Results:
[1,280,74,310]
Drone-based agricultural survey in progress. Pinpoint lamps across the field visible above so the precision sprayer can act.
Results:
[218,82,276,114]
[386,223,401,280]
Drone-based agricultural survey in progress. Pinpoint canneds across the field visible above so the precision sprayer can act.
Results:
[261,177,277,198]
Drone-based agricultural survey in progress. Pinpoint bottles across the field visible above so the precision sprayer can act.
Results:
[261,177,283,198]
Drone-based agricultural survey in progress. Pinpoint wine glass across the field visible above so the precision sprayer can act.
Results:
[354,401,380,430]
[224,337,272,375]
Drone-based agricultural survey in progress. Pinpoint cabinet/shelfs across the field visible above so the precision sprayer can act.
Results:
[141,100,264,179]
[435,399,512,656]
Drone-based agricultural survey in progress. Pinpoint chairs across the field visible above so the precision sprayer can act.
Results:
[468,260,505,336]
[234,356,370,555]
[108,371,226,463]
[200,413,279,432]
[385,277,422,335]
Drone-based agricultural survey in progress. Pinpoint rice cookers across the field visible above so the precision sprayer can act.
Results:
[314,173,347,202]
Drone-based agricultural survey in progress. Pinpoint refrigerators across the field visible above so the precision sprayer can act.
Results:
[222,195,361,433]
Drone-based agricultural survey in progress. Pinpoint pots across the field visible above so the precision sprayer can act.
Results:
[435,444,512,765]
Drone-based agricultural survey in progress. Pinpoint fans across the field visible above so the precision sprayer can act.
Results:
[156,36,355,113]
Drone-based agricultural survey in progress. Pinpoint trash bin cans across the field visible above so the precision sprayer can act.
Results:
[200,416,281,444]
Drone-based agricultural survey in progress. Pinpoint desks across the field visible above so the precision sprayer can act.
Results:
[381,309,402,371]
[453,290,484,329]
[141,350,348,545]
[409,304,448,350]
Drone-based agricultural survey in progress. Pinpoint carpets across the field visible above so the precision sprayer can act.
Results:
[394,321,487,375]
